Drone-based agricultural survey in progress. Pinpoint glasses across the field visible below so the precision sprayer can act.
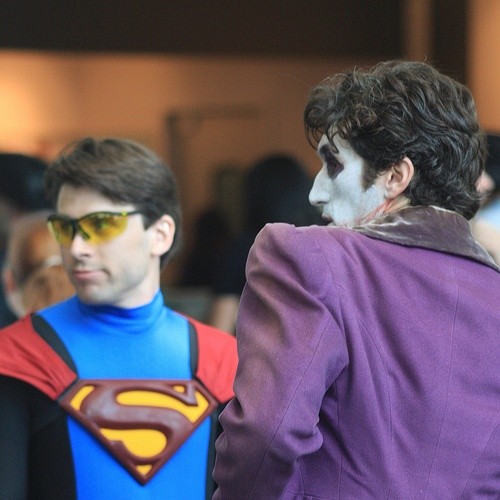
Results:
[47,210,161,247]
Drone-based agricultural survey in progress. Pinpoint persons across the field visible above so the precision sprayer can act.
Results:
[0,136,240,500]
[213,60,500,500]
[0,150,333,335]
